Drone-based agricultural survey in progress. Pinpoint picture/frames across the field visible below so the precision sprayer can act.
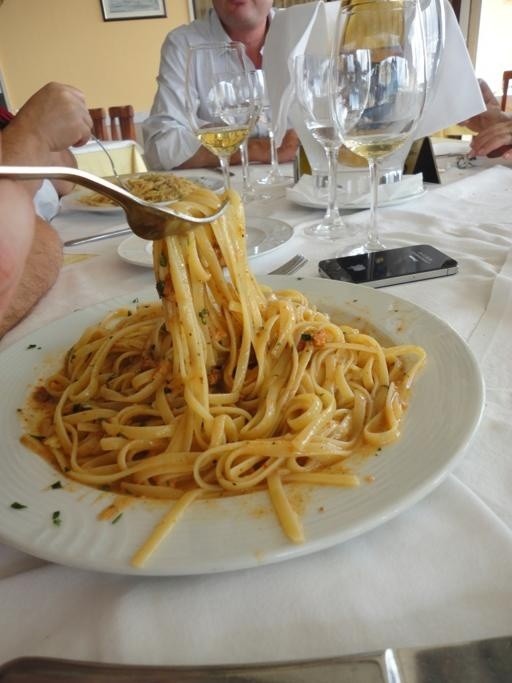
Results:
[99,0,169,22]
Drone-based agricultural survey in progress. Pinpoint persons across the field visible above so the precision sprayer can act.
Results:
[0,79,95,338]
[142,0,302,171]
[454,76,511,158]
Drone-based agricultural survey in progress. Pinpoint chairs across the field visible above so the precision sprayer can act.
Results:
[89,104,136,142]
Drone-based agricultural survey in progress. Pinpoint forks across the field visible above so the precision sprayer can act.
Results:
[1,165,230,241]
[89,135,135,194]
[269,254,309,274]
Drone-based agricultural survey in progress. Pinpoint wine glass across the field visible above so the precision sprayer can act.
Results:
[294,49,372,239]
[333,1,427,259]
[185,41,295,208]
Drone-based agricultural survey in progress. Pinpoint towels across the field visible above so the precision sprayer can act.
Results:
[261,1,488,149]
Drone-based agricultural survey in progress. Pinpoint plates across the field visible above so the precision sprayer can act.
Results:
[118,216,294,270]
[60,175,225,218]
[295,187,425,210]
[1,274,487,577]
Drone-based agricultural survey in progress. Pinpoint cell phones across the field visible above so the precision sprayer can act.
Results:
[319,242,460,288]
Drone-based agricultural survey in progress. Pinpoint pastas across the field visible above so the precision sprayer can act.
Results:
[80,172,198,208]
[22,183,426,570]
